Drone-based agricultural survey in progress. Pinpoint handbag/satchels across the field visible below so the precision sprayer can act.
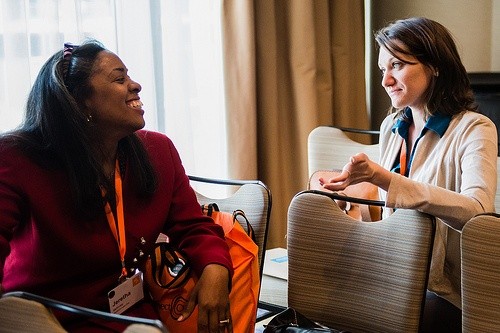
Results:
[143,202,260,332]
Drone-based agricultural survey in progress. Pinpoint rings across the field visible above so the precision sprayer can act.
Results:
[347,169,351,175]
[220,318,230,323]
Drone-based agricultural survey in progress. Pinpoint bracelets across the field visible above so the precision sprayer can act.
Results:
[339,192,351,211]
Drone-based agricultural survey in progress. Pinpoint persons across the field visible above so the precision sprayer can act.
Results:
[319,17,498,333]
[0,43,234,333]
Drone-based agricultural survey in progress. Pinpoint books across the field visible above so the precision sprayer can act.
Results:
[263,248,288,280]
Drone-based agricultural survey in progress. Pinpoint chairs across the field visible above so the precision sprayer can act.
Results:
[188,175,500,333]
[308,125,383,187]
[1,291,167,333]
